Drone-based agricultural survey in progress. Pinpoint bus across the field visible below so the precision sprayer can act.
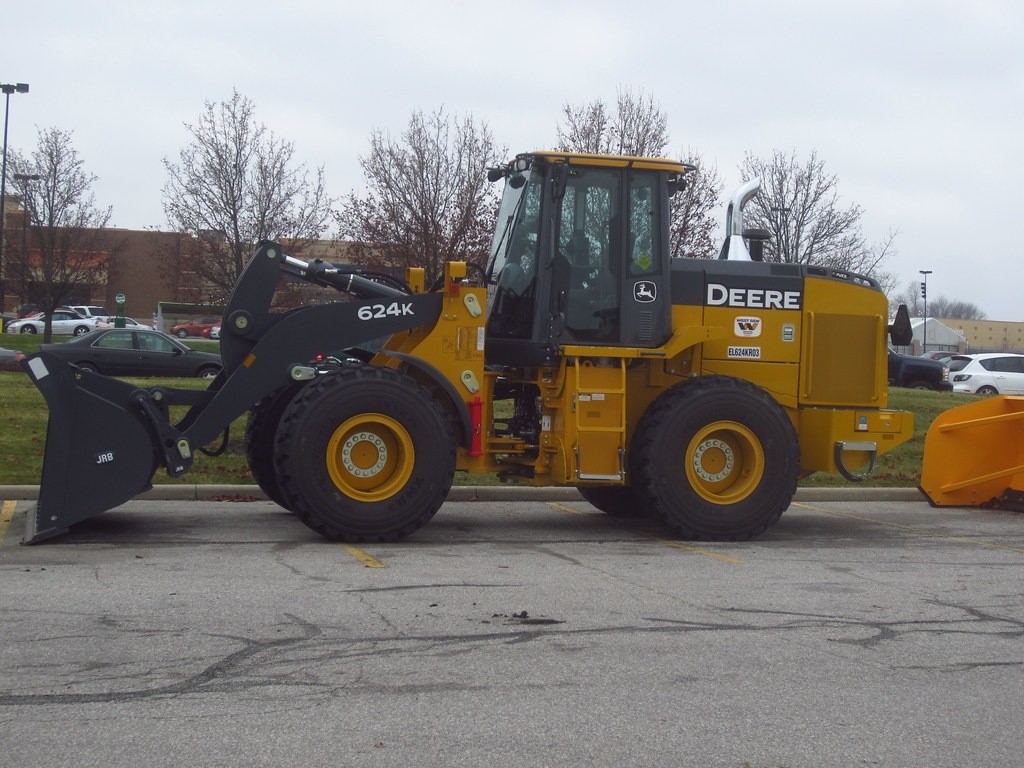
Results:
[152,301,226,334]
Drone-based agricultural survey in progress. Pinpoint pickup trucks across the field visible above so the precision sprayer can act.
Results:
[65,305,106,317]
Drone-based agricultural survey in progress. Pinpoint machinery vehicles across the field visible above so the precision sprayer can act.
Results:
[5,151,914,545]
[916,394,1024,508]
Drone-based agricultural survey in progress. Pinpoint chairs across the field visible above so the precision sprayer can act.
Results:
[591,233,637,282]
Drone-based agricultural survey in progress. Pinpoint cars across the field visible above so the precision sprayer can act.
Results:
[40,328,223,378]
[0,347,26,371]
[4,308,95,337]
[92,315,155,331]
[920,350,959,364]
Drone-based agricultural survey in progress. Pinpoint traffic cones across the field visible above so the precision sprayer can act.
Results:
[171,316,223,339]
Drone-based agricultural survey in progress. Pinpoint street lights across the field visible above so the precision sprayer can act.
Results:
[771,208,792,261]
[14,173,39,306]
[0,82,29,253]
[920,271,932,351]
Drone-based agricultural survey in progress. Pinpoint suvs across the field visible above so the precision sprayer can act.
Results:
[887,346,953,392]
[945,353,1024,397]
